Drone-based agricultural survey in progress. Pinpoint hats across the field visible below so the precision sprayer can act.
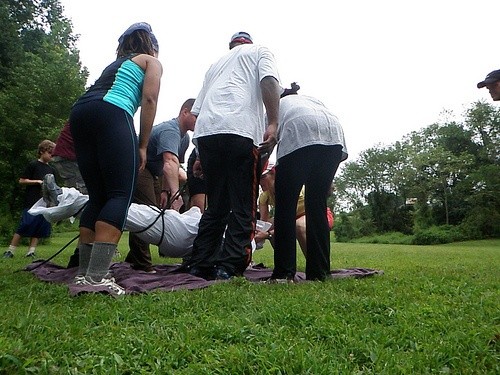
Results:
[230,31,253,44]
[476,69,500,88]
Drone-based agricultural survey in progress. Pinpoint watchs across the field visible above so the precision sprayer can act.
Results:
[267,231,273,239]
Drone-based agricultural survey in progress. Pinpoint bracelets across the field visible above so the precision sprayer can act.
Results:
[161,190,170,196]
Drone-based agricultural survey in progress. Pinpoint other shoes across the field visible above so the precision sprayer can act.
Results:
[3,250,14,258]
[26,252,35,257]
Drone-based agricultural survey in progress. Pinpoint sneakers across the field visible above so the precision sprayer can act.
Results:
[78,277,125,296]
[72,275,85,286]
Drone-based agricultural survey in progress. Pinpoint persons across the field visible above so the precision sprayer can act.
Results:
[256,83,349,283]
[68,22,164,295]
[253,159,335,261]
[182,145,209,268]
[51,122,90,269]
[191,30,282,280]
[2,139,68,258]
[27,174,276,262]
[128,98,198,271]
[477,70,500,101]
